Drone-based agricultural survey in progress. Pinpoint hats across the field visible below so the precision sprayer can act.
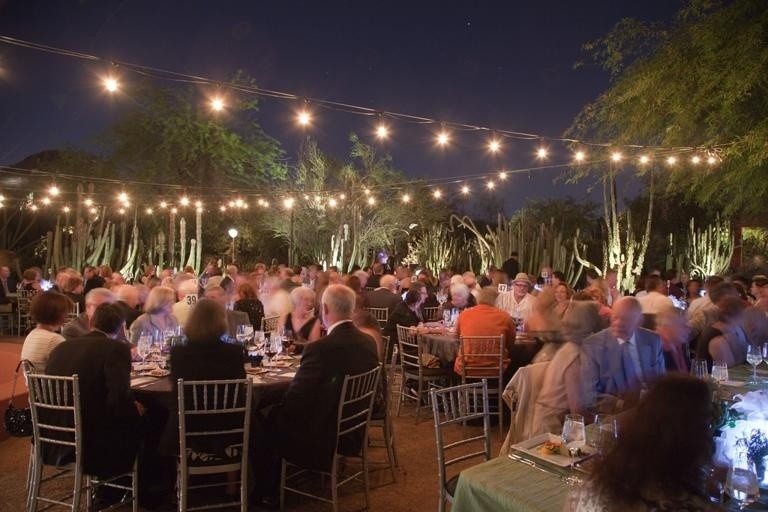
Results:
[510,273,534,287]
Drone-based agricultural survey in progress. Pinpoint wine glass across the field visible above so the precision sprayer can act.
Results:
[746,343,767,388]
[690,359,708,382]
[140,325,291,376]
[640,383,653,399]
[442,309,450,327]
[711,360,729,385]
[562,413,586,485]
[590,413,618,459]
[725,453,760,512]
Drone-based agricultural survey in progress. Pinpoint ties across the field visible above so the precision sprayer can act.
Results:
[622,342,639,390]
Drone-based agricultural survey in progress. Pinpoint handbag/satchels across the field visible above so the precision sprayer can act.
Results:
[5,407,33,436]
[417,352,440,369]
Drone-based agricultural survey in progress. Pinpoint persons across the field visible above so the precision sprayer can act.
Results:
[0,249,768,431]
[20,289,73,390]
[262,282,378,508]
[126,286,184,348]
[62,288,138,356]
[572,374,719,512]
[161,298,249,499]
[44,300,168,512]
[530,304,601,439]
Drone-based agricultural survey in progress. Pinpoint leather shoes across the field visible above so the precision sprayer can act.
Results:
[408,390,426,405]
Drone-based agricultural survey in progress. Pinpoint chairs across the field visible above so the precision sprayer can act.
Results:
[0,290,81,334]
[260,314,280,334]
[364,303,512,481]
[175,377,253,510]
[280,360,383,512]
[21,359,138,510]
[431,378,492,512]
